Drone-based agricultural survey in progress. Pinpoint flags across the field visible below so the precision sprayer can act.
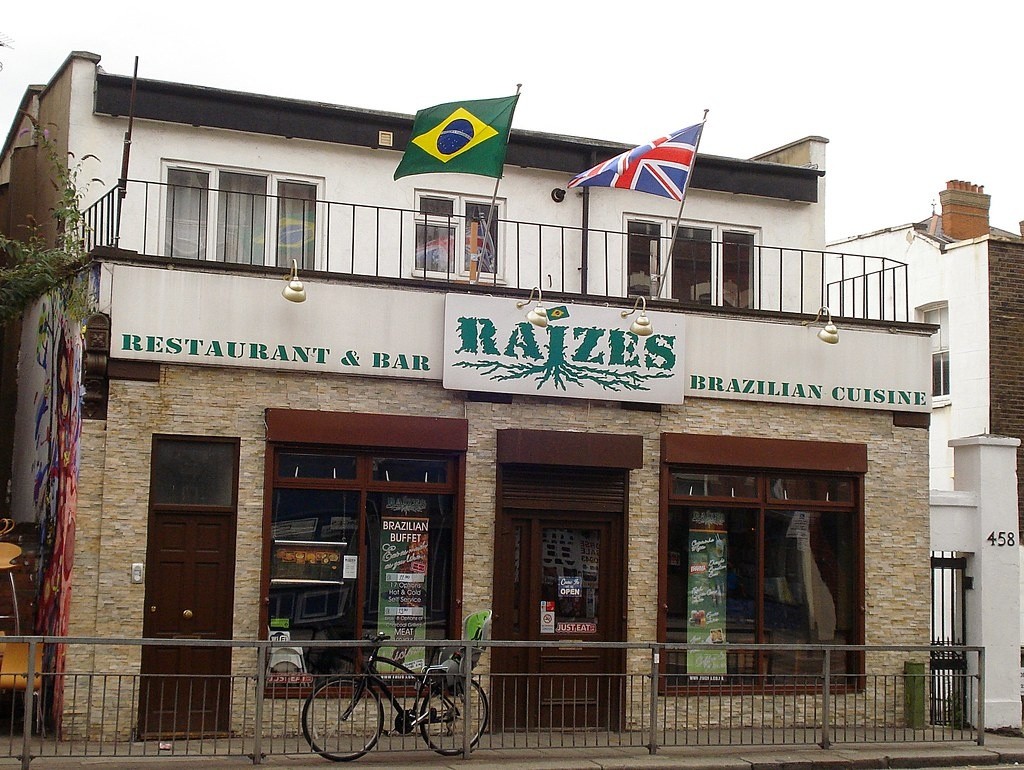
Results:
[566,119,707,201]
[243,210,314,254]
[393,95,520,181]
[416,218,495,272]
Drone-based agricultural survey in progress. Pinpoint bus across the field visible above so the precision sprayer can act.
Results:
[264,536,355,689]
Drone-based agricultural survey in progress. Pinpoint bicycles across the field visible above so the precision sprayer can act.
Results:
[300,632,489,763]
